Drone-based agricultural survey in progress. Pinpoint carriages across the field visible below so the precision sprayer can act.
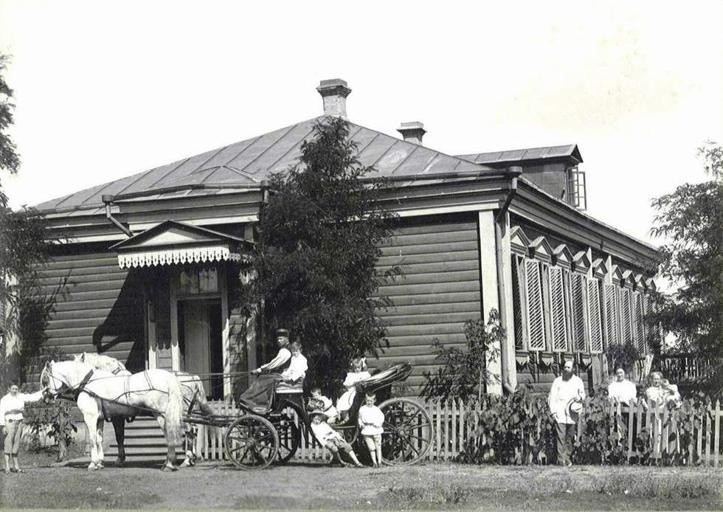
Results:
[38,349,437,473]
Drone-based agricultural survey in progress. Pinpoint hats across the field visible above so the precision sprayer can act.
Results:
[309,409,329,422]
[564,398,585,416]
[277,329,289,336]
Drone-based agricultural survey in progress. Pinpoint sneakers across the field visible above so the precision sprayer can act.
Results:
[344,462,364,467]
[374,462,384,468]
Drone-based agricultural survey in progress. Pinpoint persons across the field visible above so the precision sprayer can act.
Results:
[643,371,681,459]
[0,380,50,475]
[309,387,342,425]
[547,359,587,469]
[249,326,293,376]
[357,390,386,469]
[306,408,367,469]
[274,339,307,382]
[334,356,371,423]
[606,367,638,465]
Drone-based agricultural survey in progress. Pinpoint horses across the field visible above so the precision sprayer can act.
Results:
[37,349,212,474]
